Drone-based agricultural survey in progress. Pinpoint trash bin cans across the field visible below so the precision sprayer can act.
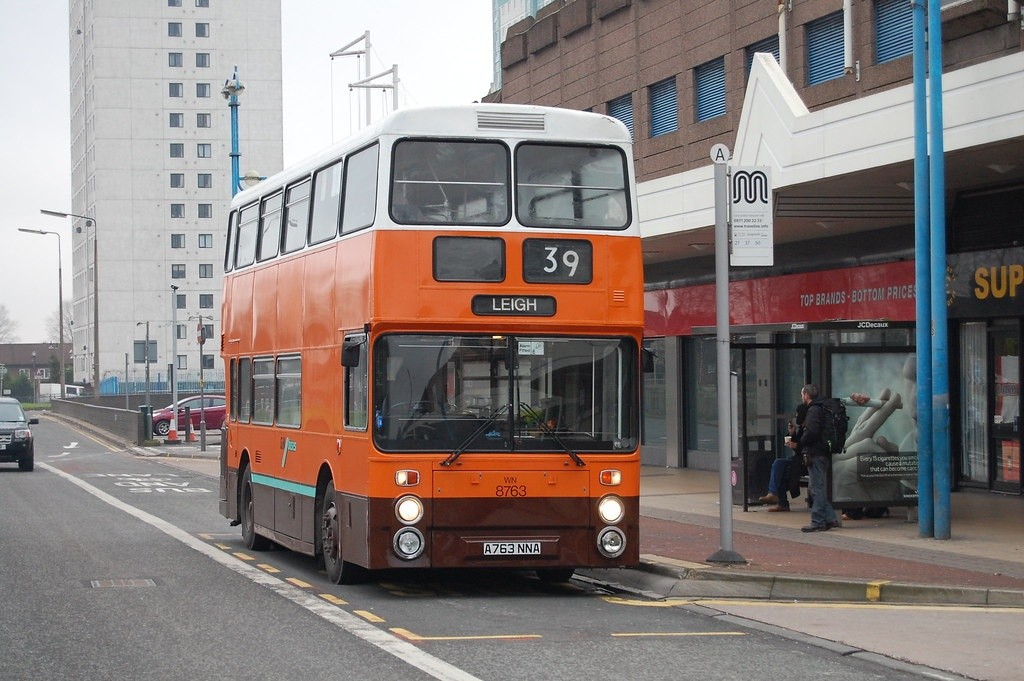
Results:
[727,433,777,506]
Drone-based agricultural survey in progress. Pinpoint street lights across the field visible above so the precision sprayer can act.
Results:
[18,227,66,398]
[187,313,215,451]
[136,320,154,442]
[41,209,103,398]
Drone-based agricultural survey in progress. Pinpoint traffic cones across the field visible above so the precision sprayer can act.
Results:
[186,419,197,442]
[164,418,182,444]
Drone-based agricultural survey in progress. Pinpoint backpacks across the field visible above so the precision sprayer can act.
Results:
[817,402,848,455]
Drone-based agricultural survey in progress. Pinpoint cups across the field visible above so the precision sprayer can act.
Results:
[784,436,792,444]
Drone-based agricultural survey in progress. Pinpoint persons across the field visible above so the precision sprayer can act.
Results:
[800,384,841,532]
[758,405,809,513]
[839,352,918,460]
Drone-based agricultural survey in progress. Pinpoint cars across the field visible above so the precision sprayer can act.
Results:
[149,394,229,436]
[0,396,39,471]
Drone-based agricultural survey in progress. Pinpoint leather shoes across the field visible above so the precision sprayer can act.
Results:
[802,525,826,532]
[769,502,789,512]
[760,492,780,503]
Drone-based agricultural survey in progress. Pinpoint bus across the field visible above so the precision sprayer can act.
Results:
[217,102,640,590]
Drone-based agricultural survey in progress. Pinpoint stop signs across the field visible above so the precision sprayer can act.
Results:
[195,322,205,344]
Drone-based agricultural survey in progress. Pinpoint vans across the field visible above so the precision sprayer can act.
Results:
[65,385,87,398]
